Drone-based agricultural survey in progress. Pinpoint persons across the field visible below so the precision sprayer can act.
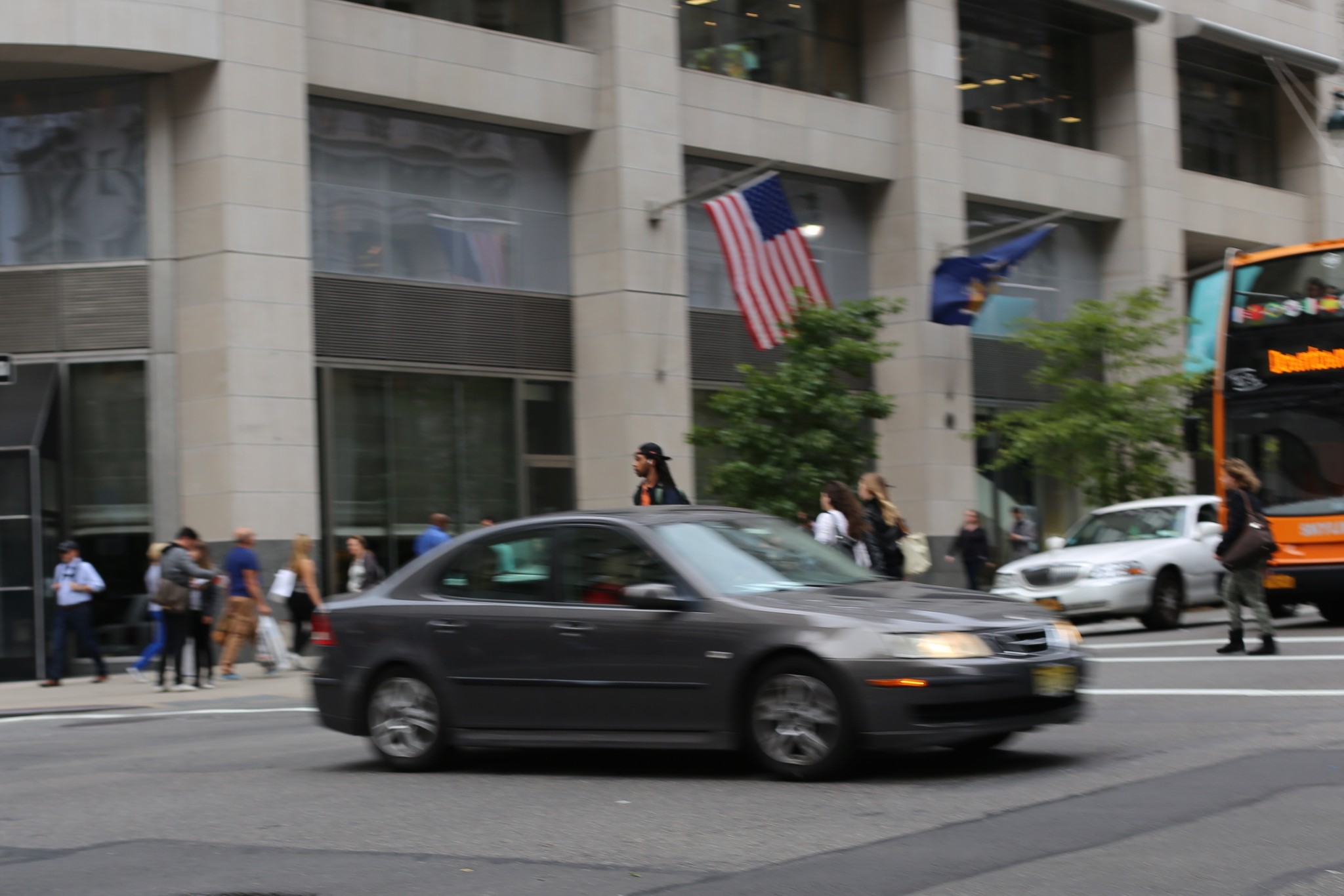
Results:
[39,540,108,687]
[797,472,912,580]
[286,535,325,671]
[944,509,997,591]
[126,526,221,693]
[582,548,645,606]
[480,515,499,528]
[1006,506,1039,559]
[216,526,278,680]
[1214,457,1280,656]
[411,513,453,558]
[632,443,682,507]
[346,533,387,594]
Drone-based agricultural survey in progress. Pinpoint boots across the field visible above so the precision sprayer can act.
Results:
[1215,628,1246,654]
[1245,635,1277,656]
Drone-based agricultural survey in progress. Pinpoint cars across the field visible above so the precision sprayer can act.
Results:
[310,508,1094,783]
[991,492,1231,632]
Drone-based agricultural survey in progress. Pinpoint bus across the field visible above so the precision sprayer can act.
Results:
[1210,238,1344,624]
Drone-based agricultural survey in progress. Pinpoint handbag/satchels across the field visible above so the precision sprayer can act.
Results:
[1220,518,1279,573]
[853,541,875,568]
[151,579,187,611]
[270,570,299,597]
[898,532,933,575]
[253,615,286,664]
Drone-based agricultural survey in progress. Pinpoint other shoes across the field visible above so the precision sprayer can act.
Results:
[152,685,170,692]
[93,674,108,683]
[127,665,146,683]
[172,683,198,692]
[220,672,243,680]
[42,680,58,687]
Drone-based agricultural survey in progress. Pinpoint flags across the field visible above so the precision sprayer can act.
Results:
[928,222,1056,330]
[703,171,837,351]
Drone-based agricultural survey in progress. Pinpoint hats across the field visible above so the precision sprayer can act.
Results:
[640,442,671,460]
[57,540,78,551]
